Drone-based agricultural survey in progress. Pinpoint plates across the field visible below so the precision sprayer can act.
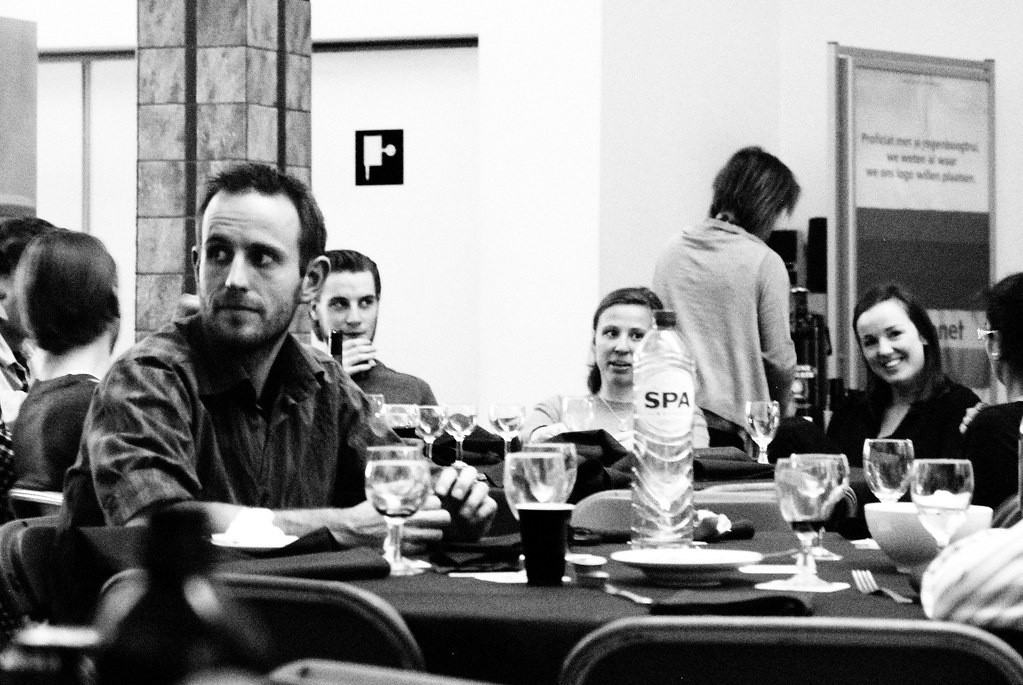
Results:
[611,550,765,588]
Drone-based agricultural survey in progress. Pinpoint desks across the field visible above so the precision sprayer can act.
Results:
[267,528,1012,685]
[482,444,902,521]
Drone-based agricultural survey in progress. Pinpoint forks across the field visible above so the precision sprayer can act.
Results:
[850,569,913,603]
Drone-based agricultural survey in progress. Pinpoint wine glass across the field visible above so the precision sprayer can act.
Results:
[364,394,524,578]
[910,458,975,556]
[746,400,779,465]
[772,453,850,593]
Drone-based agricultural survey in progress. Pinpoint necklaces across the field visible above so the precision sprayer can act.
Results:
[597,393,633,432]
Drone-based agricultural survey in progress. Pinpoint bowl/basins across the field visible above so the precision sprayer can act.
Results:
[863,502,993,574]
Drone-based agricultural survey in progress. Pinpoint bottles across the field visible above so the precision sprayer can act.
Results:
[787,288,826,412]
[630,309,695,546]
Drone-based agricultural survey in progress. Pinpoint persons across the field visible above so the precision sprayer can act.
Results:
[825,277,983,545]
[520,286,710,460]
[0,214,121,519]
[305,249,440,447]
[649,146,799,461]
[931,265,1023,628]
[55,164,495,556]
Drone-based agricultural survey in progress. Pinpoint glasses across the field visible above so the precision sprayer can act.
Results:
[977,326,1001,341]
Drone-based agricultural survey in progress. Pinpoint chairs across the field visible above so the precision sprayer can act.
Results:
[570,482,795,533]
[555,612,1023,685]
[97,564,421,668]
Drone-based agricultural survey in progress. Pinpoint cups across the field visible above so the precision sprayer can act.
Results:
[515,504,572,586]
[862,439,915,502]
[521,443,579,503]
[343,337,373,383]
[504,452,565,521]
